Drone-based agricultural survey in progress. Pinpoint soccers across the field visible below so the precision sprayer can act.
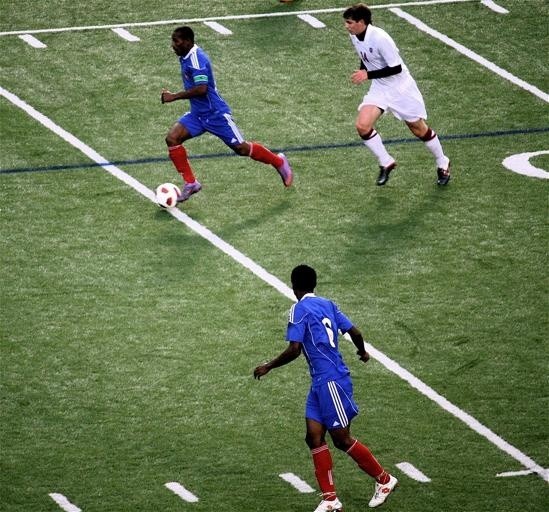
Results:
[154,182,183,210]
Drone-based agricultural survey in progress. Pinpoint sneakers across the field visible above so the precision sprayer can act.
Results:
[174,179,202,203]
[433,154,453,186]
[312,495,343,512]
[373,158,396,187]
[273,150,295,190]
[365,472,401,510]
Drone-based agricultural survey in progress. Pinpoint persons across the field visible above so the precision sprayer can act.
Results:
[253,264,399,512]
[161,26,292,202]
[344,2,452,186]
